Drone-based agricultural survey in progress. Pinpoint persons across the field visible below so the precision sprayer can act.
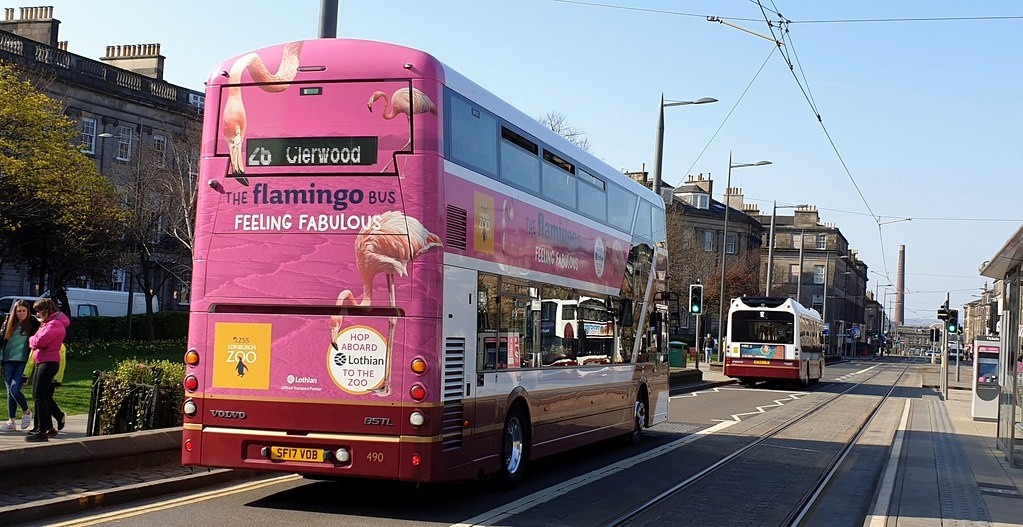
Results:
[0,297,40,431]
[21,297,70,442]
[701,333,714,363]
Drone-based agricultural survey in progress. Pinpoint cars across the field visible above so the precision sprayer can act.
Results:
[925,350,933,356]
[935,352,939,355]
[713,339,718,350]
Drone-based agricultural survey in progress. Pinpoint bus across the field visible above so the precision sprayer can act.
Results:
[726,294,829,387]
[180,40,681,487]
[522,296,615,362]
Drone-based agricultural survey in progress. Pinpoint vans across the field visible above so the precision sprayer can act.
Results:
[0,296,100,317]
[949,344,964,359]
[39,287,159,317]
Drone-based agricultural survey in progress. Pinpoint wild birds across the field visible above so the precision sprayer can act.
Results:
[221,41,303,186]
[367,86,438,179]
[329,211,443,397]
[603,239,627,291]
[500,197,514,254]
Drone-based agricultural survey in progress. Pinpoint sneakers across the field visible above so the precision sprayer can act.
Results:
[28,428,40,435]
[0,422,17,432]
[26,433,48,442]
[21,410,32,429]
[45,428,58,437]
[58,411,66,430]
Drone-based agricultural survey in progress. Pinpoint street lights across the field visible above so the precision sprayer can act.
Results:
[652,96,717,196]
[718,161,774,361]
[822,256,849,323]
[882,293,899,333]
[889,301,901,331]
[766,205,808,297]
[98,133,140,231]
[797,232,828,303]
[876,285,892,301]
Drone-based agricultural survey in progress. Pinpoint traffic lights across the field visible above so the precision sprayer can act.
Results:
[689,284,703,316]
[947,309,958,334]
[938,300,949,321]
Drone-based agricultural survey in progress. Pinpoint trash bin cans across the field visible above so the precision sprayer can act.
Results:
[668,341,689,369]
